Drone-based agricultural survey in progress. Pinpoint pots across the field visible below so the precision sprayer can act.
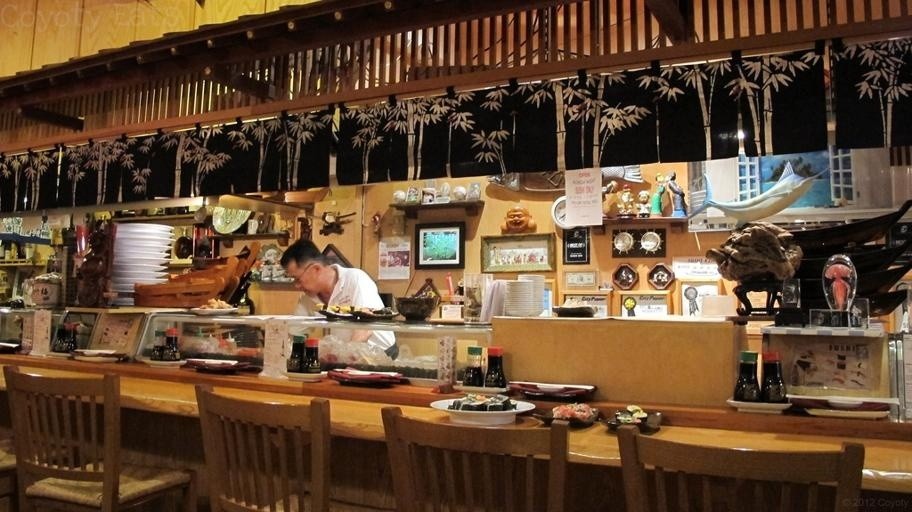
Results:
[30,272,77,310]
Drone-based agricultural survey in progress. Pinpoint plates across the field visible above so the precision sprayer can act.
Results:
[280,371,326,385]
[690,223,706,230]
[75,348,117,355]
[553,304,600,317]
[803,407,891,419]
[186,358,238,368]
[430,395,539,428]
[726,397,792,417]
[190,307,238,316]
[209,359,240,366]
[137,355,187,368]
[195,366,240,374]
[609,412,660,434]
[109,224,176,307]
[329,369,408,388]
[73,355,126,364]
[512,378,599,403]
[504,280,534,317]
[830,396,867,408]
[518,274,546,318]
[456,381,511,395]
[539,384,564,393]
[404,377,460,387]
[536,405,600,429]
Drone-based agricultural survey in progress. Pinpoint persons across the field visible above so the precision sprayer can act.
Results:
[665,172,687,217]
[635,190,653,218]
[278,238,400,362]
[649,173,666,218]
[500,205,538,235]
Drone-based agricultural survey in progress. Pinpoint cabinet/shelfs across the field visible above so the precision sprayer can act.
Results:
[134,313,493,387]
[0,231,53,307]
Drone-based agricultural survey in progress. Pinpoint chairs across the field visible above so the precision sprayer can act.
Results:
[2,364,193,511]
[615,425,866,512]
[0,432,70,512]
[382,407,570,512]
[193,381,356,512]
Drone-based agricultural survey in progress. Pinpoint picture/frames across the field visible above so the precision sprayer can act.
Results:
[678,279,724,317]
[617,291,674,318]
[647,263,674,291]
[612,263,639,290]
[561,290,612,318]
[481,232,556,274]
[415,221,465,269]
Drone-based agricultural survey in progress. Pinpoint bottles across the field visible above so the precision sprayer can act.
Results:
[286,334,307,373]
[484,346,508,389]
[161,327,181,362]
[237,283,254,315]
[302,338,321,374]
[462,345,484,389]
[149,334,162,362]
[53,329,64,352]
[760,350,785,402]
[63,327,76,351]
[732,349,761,404]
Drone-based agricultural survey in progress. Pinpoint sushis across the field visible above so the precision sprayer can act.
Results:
[445,392,520,415]
[612,402,649,427]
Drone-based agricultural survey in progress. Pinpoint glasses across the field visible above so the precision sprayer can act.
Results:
[288,262,313,286]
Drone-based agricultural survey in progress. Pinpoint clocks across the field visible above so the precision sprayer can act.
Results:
[552,195,578,229]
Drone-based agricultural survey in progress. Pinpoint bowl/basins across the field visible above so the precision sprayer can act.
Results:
[689,190,707,210]
[395,296,439,322]
